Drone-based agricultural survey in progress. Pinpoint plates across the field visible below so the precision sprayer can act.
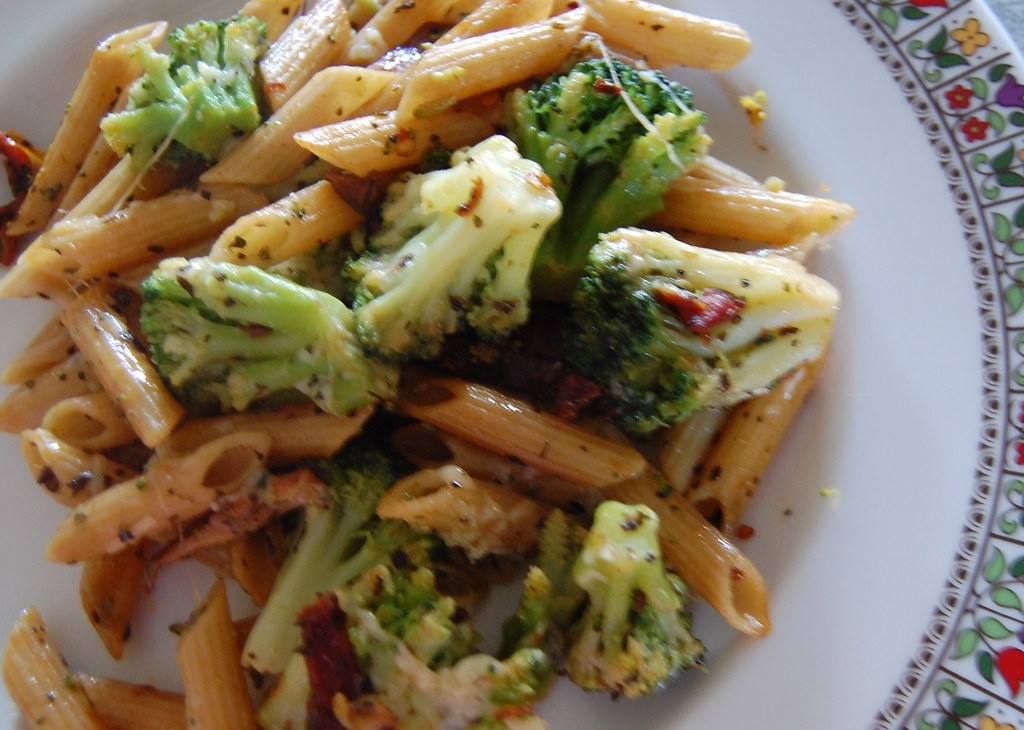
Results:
[0,0,1024,730]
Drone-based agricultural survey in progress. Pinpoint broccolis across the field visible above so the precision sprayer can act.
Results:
[93,13,839,730]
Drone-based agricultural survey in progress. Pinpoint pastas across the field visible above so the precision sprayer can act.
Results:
[0,2,854,730]
